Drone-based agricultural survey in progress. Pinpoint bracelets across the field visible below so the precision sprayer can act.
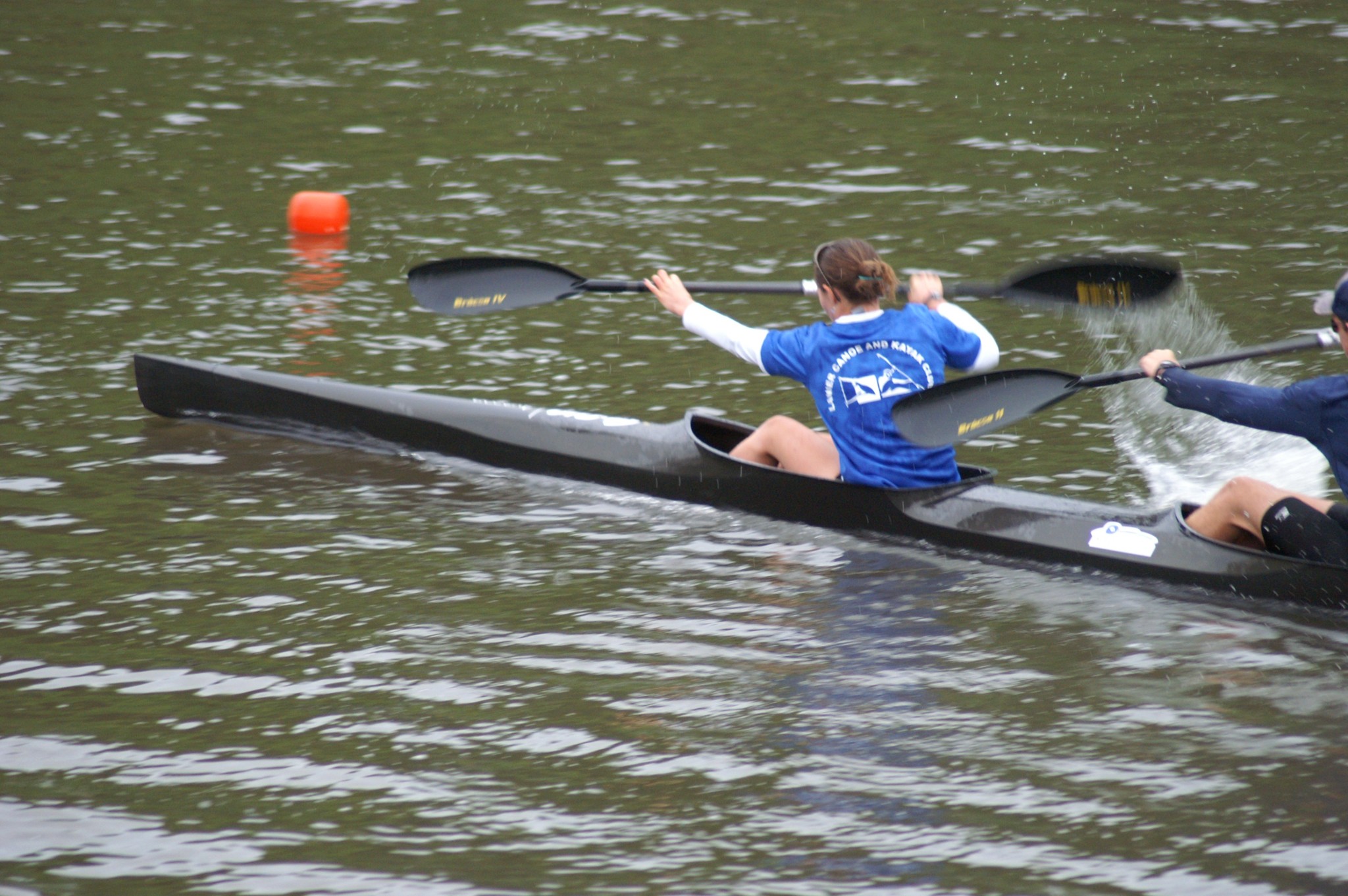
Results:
[922,292,945,309]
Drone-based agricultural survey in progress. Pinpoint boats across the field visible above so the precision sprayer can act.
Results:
[132,351,1348,613]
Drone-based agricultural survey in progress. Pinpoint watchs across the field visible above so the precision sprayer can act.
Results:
[1153,360,1182,380]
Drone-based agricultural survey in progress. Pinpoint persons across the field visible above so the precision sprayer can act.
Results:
[643,236,1003,489]
[1137,271,1348,567]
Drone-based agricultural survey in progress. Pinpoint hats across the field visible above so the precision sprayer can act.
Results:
[1332,273,1348,320]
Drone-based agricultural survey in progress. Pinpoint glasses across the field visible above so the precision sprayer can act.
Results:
[813,241,841,302]
[1330,319,1339,333]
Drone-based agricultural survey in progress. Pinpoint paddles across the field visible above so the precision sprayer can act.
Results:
[887,325,1347,451]
[403,244,1187,323]
